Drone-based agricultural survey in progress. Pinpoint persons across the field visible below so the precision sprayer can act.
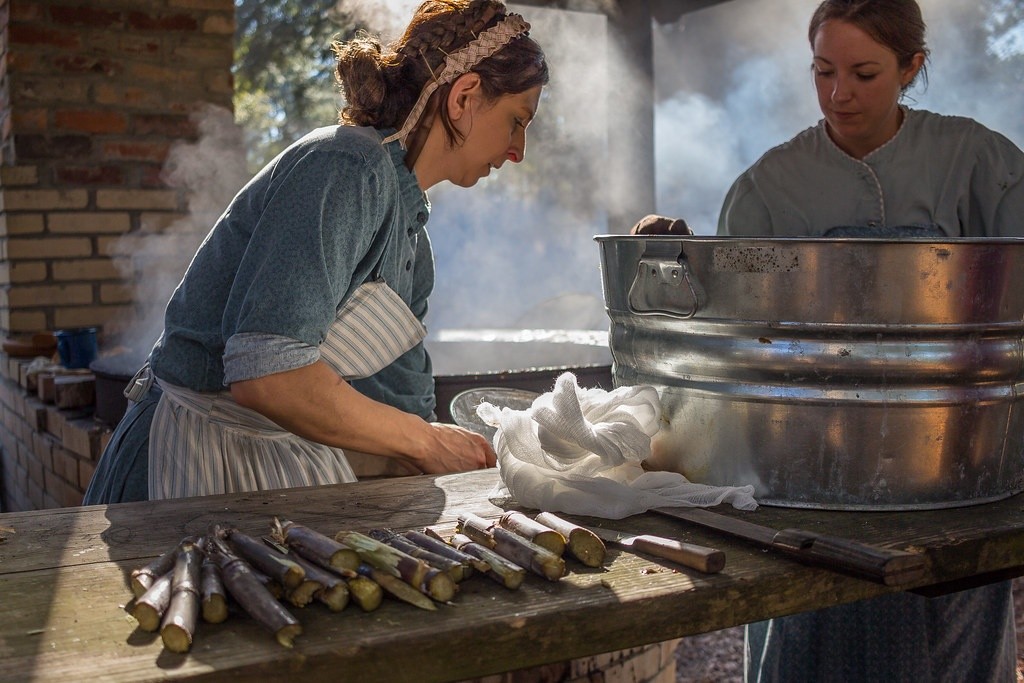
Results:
[633,0,1023,683]
[81,1,548,506]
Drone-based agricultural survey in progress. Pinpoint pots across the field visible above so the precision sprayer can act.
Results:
[89,353,151,425]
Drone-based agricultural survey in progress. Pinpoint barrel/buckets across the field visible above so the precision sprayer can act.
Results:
[595,233,1022,512]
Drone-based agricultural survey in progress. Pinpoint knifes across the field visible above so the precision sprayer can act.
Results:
[652,505,924,587]
[581,526,726,574]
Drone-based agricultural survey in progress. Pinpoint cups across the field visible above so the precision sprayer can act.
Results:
[54,327,97,369]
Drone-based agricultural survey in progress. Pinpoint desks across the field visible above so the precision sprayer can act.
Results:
[0,466,1024,683]
